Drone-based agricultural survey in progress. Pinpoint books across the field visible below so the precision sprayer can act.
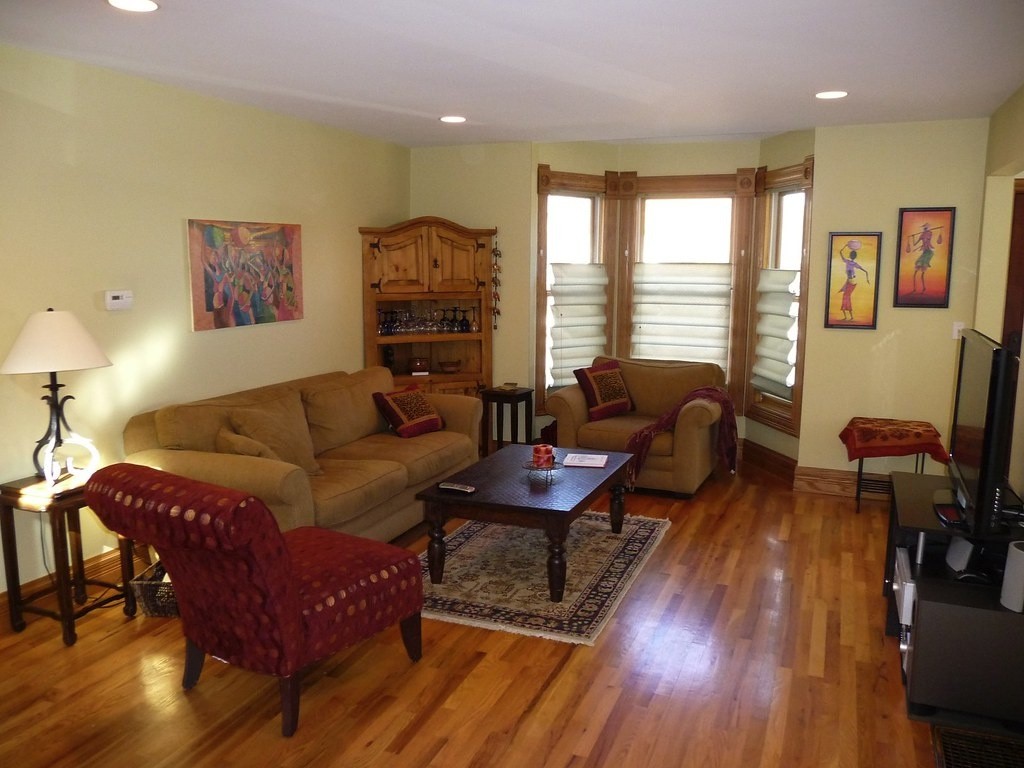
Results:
[563,453,608,467]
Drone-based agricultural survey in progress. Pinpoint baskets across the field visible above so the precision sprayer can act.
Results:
[129,558,180,618]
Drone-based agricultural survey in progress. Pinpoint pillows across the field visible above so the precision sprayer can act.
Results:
[216,428,280,461]
[573,360,636,422]
[372,382,447,439]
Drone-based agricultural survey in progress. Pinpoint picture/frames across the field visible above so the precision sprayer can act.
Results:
[893,207,955,309]
[824,231,882,329]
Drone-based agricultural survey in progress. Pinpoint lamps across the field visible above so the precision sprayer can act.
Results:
[1,306,112,487]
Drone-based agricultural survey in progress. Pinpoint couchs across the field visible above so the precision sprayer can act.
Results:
[122,365,483,545]
[544,353,726,501]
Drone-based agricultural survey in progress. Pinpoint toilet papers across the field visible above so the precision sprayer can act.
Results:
[1000,541,1024,613]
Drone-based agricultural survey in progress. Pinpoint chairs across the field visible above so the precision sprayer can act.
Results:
[83,463,427,738]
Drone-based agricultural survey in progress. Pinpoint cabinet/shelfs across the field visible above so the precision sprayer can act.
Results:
[359,216,498,452]
[882,472,1024,741]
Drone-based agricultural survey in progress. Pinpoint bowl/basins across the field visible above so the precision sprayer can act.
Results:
[438,361,460,371]
[408,358,428,371]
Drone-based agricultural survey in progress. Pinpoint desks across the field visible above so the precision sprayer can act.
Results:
[480,386,534,458]
[839,417,949,513]
[1,467,136,644]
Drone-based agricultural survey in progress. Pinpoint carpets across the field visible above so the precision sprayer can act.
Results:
[417,508,673,647]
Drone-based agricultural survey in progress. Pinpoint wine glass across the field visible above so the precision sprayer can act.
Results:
[376,307,479,335]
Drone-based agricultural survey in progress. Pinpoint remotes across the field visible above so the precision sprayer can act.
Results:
[438,482,475,495]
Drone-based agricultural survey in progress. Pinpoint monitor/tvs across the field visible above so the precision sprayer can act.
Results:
[934,328,1021,539]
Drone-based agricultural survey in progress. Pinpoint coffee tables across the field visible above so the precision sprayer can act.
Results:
[415,443,635,603]
